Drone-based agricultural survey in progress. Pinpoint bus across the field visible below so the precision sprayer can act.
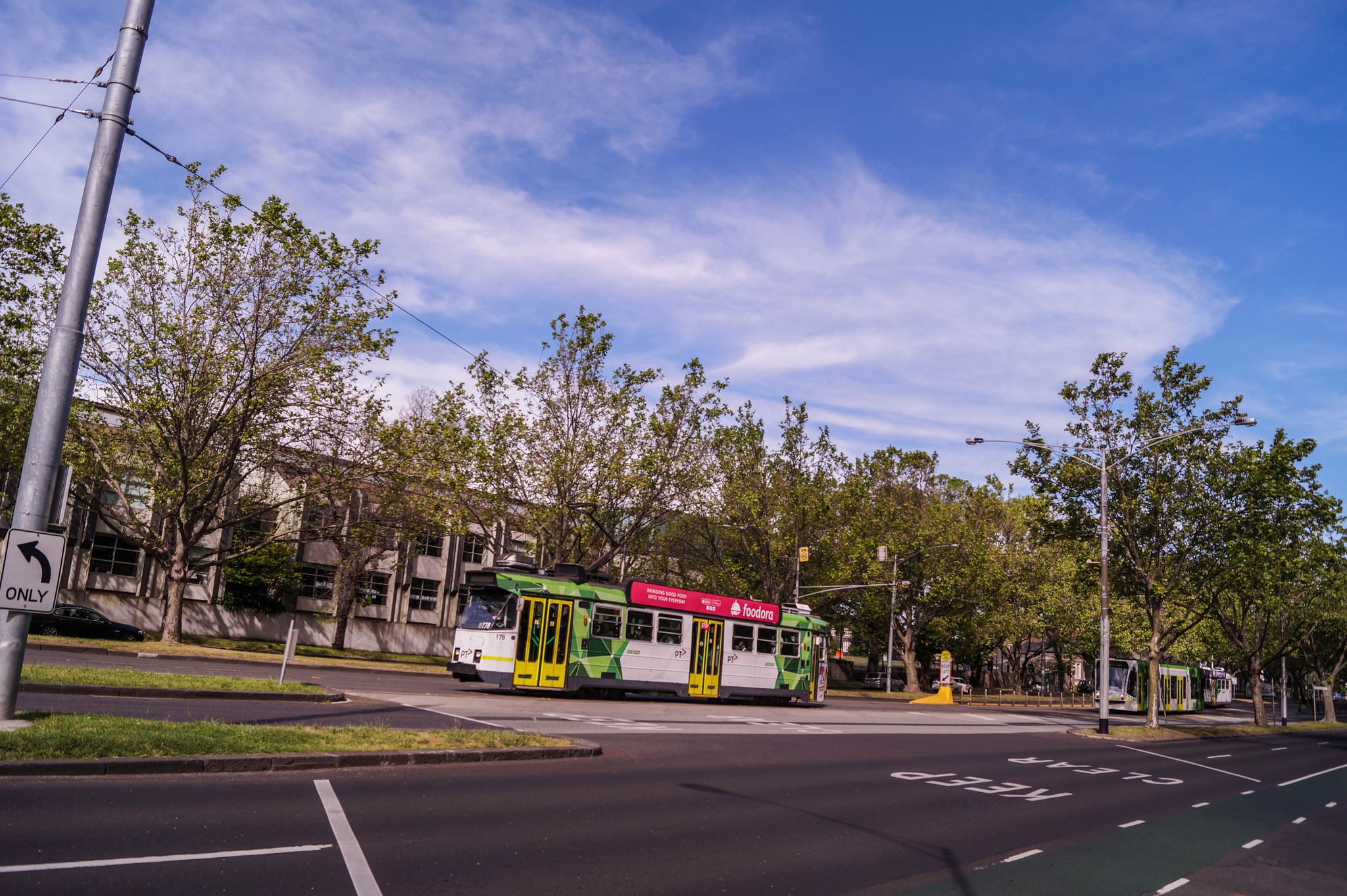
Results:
[1092,658,1204,714]
[447,552,832,708]
[1200,665,1233,707]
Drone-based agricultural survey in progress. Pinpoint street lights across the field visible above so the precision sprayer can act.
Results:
[884,543,959,693]
[965,417,1257,735]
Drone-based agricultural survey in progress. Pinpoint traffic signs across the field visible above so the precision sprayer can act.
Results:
[0,527,68,615]
[940,663,951,684]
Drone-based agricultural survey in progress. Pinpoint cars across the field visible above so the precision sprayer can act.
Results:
[29,604,146,642]
[1332,693,1346,702]
[1245,681,1276,700]
[933,676,973,694]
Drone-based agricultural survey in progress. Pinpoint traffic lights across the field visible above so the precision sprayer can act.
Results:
[987,661,992,671]
[1028,663,1035,673]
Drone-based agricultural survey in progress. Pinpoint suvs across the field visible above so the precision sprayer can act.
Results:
[1028,680,1050,694]
[864,672,905,691]
[1077,679,1095,693]
